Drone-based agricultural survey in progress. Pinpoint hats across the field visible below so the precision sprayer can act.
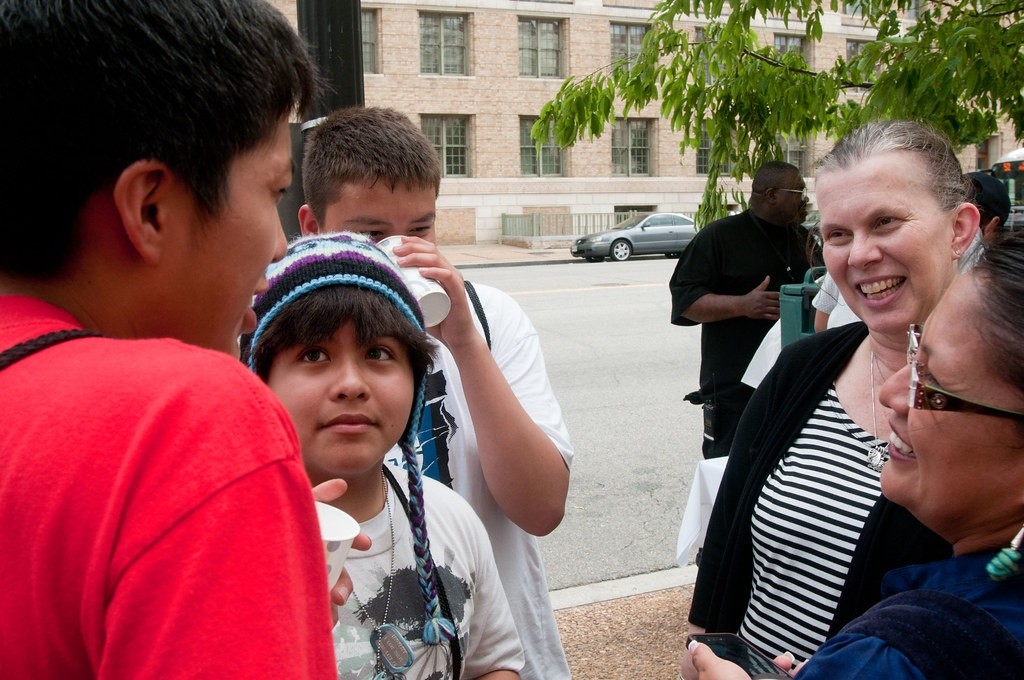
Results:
[240,231,455,643]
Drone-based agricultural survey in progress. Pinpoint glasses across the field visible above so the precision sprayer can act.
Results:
[905,323,1023,425]
[776,187,807,201]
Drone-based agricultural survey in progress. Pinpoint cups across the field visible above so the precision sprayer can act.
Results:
[315,501,361,591]
[376,234,451,329]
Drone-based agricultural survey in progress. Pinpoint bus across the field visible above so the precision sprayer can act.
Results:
[976,147,1024,230]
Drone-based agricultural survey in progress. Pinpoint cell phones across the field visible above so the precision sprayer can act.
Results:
[686,633,795,680]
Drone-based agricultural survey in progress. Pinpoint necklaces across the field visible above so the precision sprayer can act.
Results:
[352,468,415,680]
[868,351,891,474]
[748,209,795,282]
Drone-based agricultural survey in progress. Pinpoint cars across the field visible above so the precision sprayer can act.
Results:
[800,211,824,247]
[569,212,700,263]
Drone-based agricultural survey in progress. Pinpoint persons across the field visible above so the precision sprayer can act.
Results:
[688,231,1024,679]
[811,171,1010,332]
[0,0,339,680]
[298,108,573,679]
[669,161,826,459]
[237,231,525,680]
[682,117,980,671]
